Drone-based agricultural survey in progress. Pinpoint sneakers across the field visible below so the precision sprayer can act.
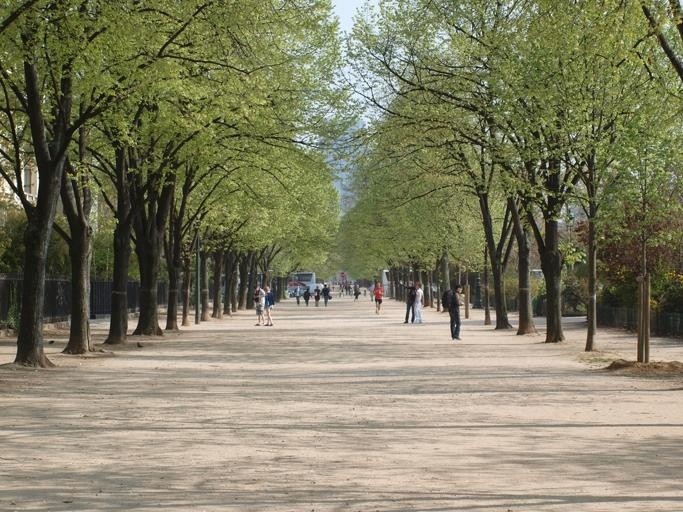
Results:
[256,323,273,326]
[454,336,462,340]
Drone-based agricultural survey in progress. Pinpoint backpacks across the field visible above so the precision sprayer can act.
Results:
[442,289,453,308]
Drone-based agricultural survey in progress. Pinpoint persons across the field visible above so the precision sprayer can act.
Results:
[369,285,375,301]
[398,281,424,323]
[322,284,330,307]
[373,282,384,314]
[304,289,311,305]
[314,286,320,306]
[294,285,300,304]
[252,285,265,326]
[264,286,275,326]
[448,284,463,340]
[338,282,361,301]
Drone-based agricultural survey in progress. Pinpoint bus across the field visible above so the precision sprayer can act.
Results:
[209,271,317,298]
[382,270,441,299]
